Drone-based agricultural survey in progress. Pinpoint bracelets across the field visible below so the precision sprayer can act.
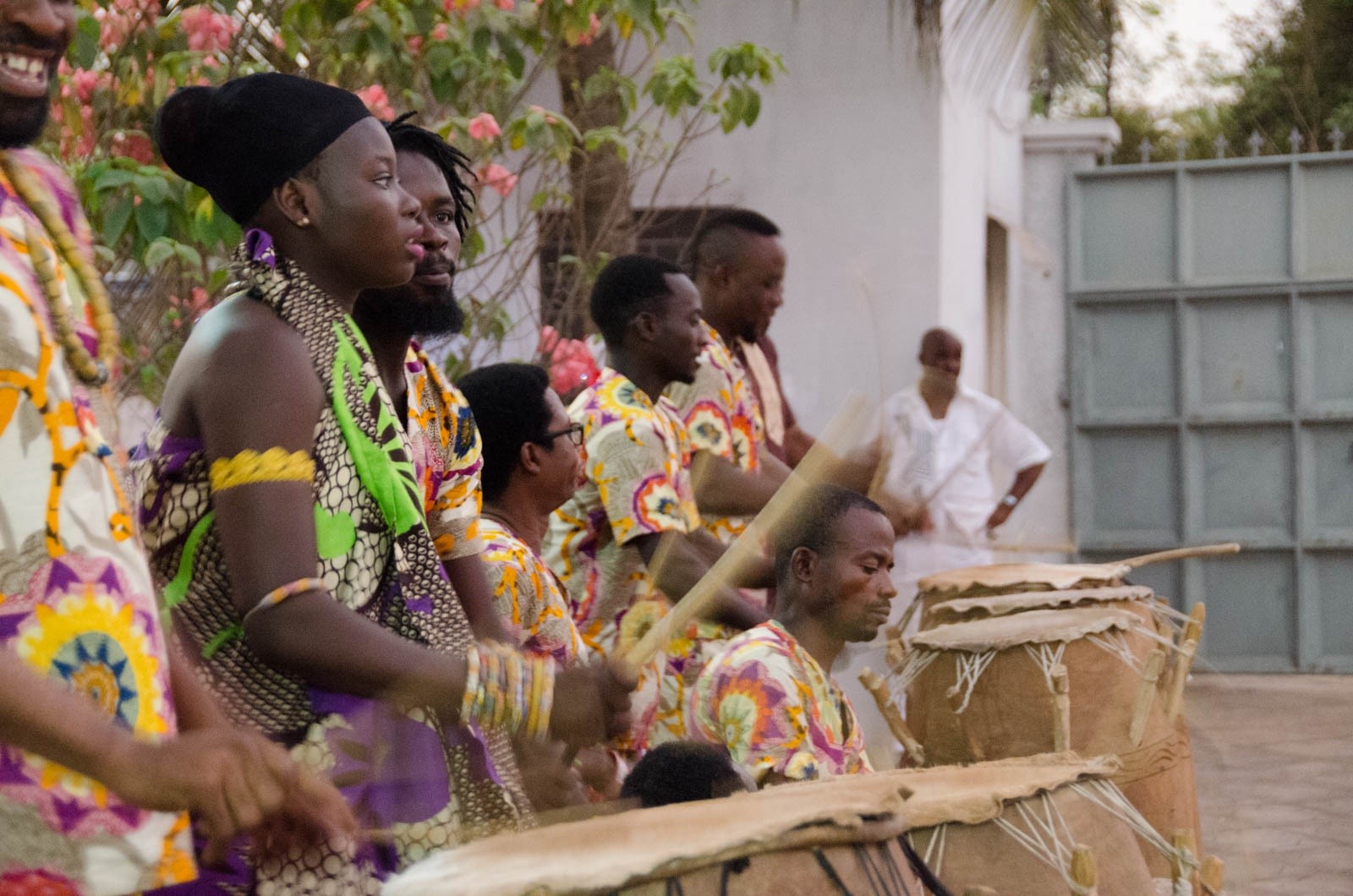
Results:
[591,750,628,800]
[1003,494,1018,507]
[458,641,557,745]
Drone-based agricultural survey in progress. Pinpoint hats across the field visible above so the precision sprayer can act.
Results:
[159,74,370,233]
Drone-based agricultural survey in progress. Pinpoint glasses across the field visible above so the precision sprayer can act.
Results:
[519,422,585,474]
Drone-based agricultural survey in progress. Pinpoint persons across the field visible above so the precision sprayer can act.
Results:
[616,740,748,815]
[137,72,642,896]
[732,332,840,488]
[0,2,359,896]
[350,107,520,650]
[661,205,919,616]
[448,362,629,824]
[540,250,897,790]
[864,326,1053,639]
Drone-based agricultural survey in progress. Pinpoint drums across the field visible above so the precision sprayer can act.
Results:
[885,597,1206,769]
[382,775,925,896]
[860,601,1203,878]
[887,561,1168,651]
[760,750,1225,896]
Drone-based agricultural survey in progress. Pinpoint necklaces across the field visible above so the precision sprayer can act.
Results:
[0,147,114,385]
